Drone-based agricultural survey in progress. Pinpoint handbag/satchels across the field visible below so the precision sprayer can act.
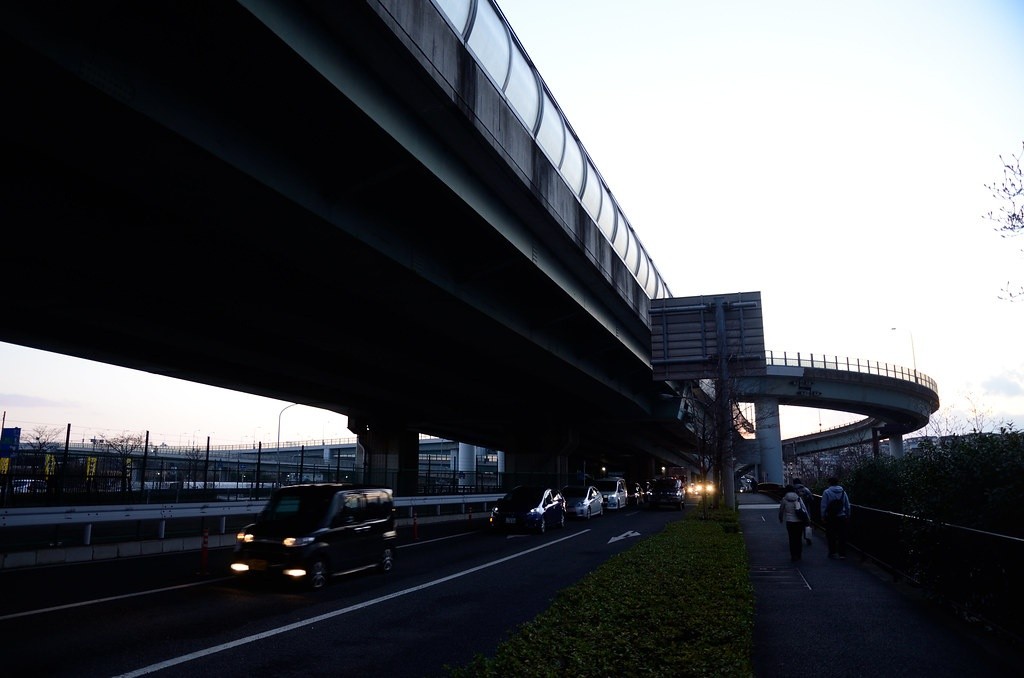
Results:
[826,490,845,515]
[795,498,809,523]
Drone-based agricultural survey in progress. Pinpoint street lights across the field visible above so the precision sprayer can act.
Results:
[892,327,919,383]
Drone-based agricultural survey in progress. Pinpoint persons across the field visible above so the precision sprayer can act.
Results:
[779,477,813,563]
[820,477,850,559]
[750,478,758,495]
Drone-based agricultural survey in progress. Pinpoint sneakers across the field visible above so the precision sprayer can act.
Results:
[828,552,837,559]
[839,554,849,559]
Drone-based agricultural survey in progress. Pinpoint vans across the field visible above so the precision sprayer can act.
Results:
[236,483,401,587]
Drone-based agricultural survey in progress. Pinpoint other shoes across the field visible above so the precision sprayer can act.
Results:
[805,540,812,545]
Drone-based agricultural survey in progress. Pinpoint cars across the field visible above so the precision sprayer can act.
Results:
[592,477,629,513]
[626,483,643,507]
[562,486,604,521]
[490,485,567,538]
[646,477,714,512]
[13,480,46,493]
